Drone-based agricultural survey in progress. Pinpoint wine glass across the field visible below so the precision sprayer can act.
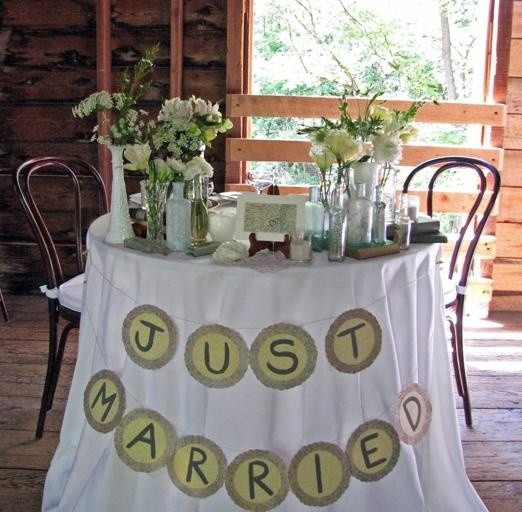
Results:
[206,181,214,197]
[245,170,275,195]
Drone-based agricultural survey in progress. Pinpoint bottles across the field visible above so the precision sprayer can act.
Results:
[165,181,191,252]
[304,184,412,262]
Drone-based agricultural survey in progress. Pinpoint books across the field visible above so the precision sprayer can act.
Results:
[381,209,451,246]
[317,232,400,259]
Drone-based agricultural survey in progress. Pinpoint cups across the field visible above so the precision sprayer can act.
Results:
[398,195,421,221]
[288,231,313,264]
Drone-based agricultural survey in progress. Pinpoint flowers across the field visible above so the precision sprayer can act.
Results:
[77,43,233,240]
[296,88,425,196]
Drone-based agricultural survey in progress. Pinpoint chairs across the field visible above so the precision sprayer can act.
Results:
[16,156,109,438]
[402,156,501,426]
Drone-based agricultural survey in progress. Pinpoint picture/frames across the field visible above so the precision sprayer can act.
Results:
[234,195,306,242]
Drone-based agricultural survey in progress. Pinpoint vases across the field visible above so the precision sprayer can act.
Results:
[108,145,211,253]
[323,163,388,261]
